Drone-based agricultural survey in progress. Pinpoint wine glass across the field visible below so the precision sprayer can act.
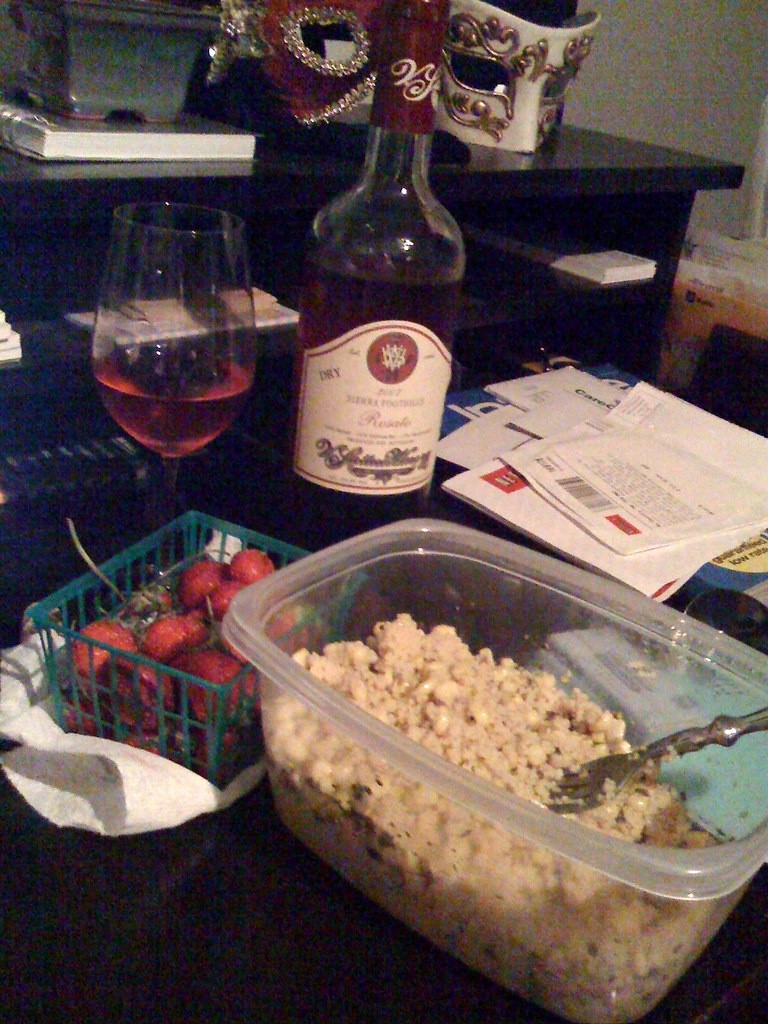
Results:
[91,199,256,526]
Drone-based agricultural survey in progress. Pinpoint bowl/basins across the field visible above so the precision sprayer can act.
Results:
[224,518,768,1023]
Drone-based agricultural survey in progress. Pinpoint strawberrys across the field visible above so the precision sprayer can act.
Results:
[65,548,324,787]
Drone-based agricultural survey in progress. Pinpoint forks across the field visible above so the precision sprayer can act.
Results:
[547,703,768,814]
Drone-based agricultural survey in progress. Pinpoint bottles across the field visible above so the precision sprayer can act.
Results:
[283,0,466,553]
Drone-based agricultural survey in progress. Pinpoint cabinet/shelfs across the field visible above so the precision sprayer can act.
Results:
[1,125,746,622]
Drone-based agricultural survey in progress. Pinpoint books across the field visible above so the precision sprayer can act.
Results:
[67,285,300,346]
[0,95,265,162]
[1,311,23,363]
[535,629,768,860]
[438,363,768,606]
[490,226,656,284]
